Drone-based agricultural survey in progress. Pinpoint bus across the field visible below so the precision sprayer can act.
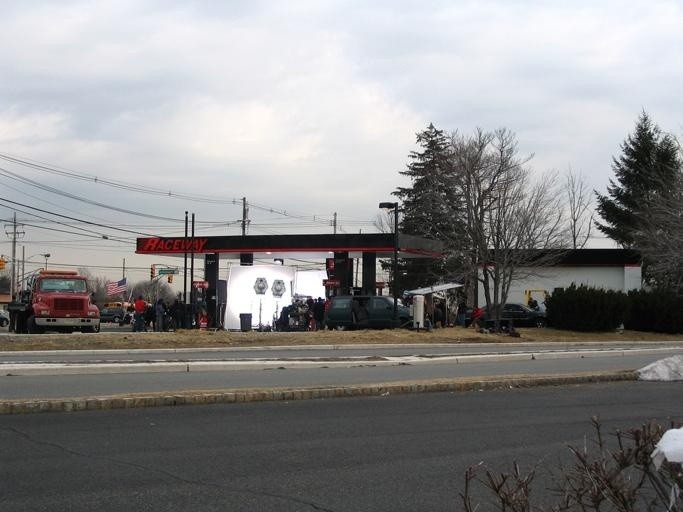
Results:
[104,302,152,313]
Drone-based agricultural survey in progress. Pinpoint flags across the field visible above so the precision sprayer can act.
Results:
[106,279,126,297]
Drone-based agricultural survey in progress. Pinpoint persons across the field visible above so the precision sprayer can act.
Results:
[527,297,539,310]
[277,295,332,331]
[425,294,480,330]
[124,287,184,332]
[540,295,552,312]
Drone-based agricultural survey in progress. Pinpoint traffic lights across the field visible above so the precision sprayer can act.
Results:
[150,265,155,278]
[167,274,173,283]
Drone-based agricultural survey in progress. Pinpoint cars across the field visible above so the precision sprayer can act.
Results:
[464,302,549,329]
[0,311,10,327]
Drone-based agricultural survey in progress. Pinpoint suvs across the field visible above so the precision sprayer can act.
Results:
[97,306,130,323]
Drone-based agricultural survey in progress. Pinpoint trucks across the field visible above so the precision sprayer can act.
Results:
[10,270,101,336]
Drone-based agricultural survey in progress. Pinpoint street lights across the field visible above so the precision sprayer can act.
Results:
[22,246,50,296]
[379,202,398,311]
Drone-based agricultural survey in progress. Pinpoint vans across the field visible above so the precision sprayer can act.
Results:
[324,296,411,332]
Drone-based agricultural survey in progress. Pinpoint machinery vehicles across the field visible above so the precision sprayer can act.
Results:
[524,289,550,309]
